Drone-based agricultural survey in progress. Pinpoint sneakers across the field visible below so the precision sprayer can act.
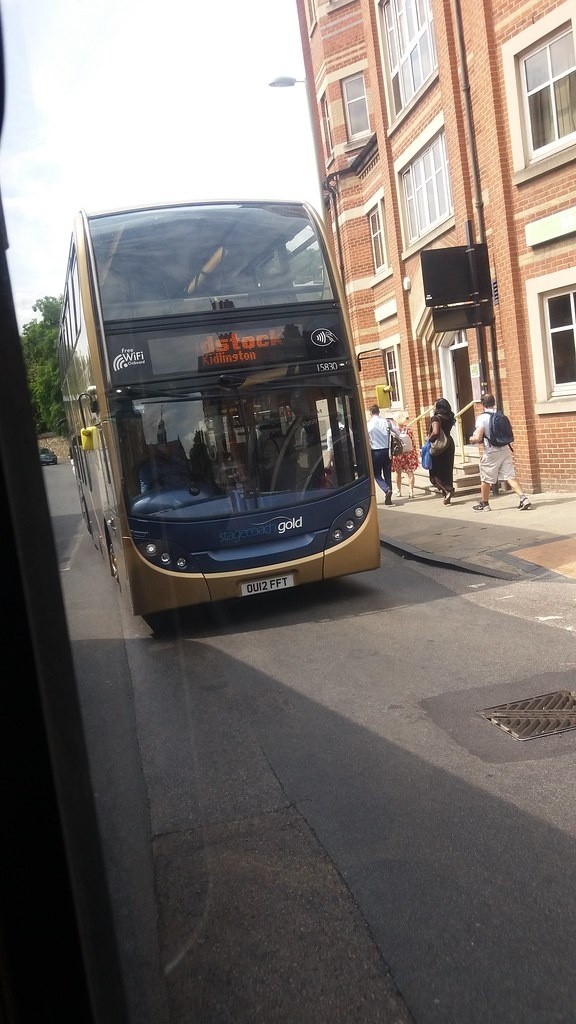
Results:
[516,498,531,510]
[472,500,491,512]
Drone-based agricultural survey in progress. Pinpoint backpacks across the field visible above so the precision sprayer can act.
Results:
[395,427,413,453]
[481,411,514,448]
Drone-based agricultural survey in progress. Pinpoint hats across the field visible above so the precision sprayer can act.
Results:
[393,411,406,425]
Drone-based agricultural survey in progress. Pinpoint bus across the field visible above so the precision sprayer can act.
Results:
[54,196,399,620]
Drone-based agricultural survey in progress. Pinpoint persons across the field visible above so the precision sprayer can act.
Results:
[389,411,418,500]
[138,430,190,494]
[366,405,402,507]
[425,398,456,505]
[327,412,357,469]
[469,394,531,511]
[258,419,295,488]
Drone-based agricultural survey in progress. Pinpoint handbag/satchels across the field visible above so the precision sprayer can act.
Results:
[386,420,403,456]
[428,415,450,457]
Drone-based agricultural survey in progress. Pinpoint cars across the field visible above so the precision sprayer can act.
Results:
[39,448,58,465]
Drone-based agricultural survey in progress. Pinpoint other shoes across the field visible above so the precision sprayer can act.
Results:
[443,489,455,505]
[408,493,414,497]
[385,489,393,505]
[395,492,401,497]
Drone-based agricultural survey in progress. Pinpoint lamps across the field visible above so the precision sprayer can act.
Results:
[270,77,306,87]
